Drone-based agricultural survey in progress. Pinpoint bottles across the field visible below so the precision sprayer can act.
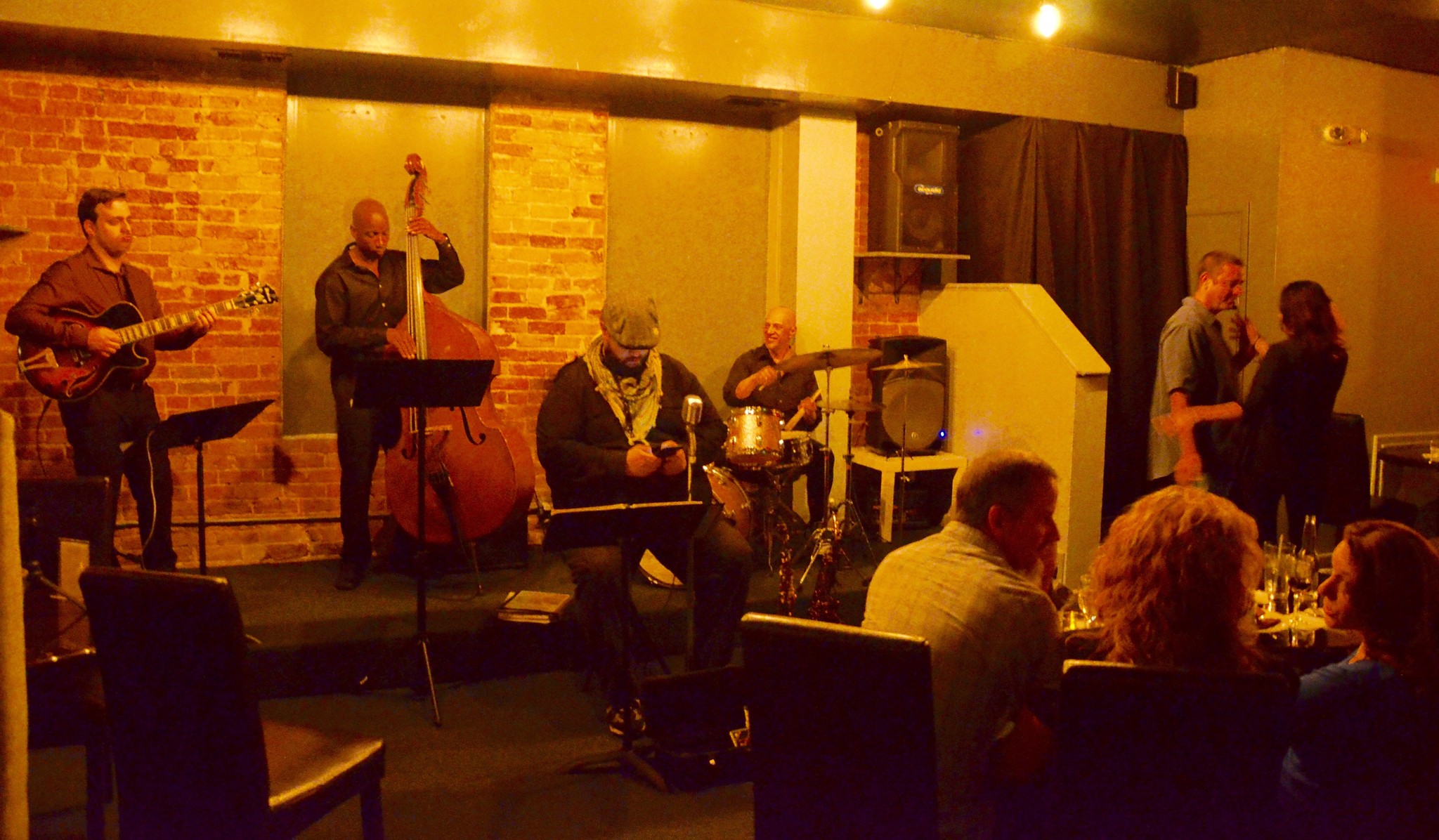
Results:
[1298,515,1320,592]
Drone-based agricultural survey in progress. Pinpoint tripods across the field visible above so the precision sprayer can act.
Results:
[790,352,877,591]
[348,355,494,728]
[536,501,710,790]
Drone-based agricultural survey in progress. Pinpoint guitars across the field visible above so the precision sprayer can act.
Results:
[18,285,282,401]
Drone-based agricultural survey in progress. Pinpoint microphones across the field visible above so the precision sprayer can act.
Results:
[682,394,702,465]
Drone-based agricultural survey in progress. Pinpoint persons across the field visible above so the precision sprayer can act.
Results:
[1278,519,1439,840]
[1150,249,1259,493]
[1151,281,1350,548]
[314,198,465,593]
[862,448,1073,840]
[5,188,218,572]
[722,306,835,561]
[534,287,749,750]
[993,484,1302,787]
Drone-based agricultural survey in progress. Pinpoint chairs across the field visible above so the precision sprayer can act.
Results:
[736,608,940,840]
[1303,404,1418,561]
[1041,652,1299,840]
[76,563,388,840]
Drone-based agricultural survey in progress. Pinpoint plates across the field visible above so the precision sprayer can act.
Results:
[1286,610,1326,631]
[1256,610,1290,633]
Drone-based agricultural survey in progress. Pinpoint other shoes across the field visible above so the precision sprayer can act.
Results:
[605,698,647,735]
[373,534,415,575]
[333,557,364,590]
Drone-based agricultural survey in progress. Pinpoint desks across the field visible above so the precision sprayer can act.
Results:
[1374,444,1439,471]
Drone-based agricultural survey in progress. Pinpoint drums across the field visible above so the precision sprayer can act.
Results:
[724,407,783,465]
[637,464,750,590]
[736,432,812,469]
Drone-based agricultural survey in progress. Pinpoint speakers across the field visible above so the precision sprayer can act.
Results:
[865,335,948,458]
[1166,71,1197,109]
[867,120,962,255]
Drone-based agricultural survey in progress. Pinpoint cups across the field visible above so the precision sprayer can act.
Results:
[1263,541,1296,601]
[1291,593,1317,647]
[1078,575,1098,620]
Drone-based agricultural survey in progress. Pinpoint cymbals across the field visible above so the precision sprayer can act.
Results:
[867,359,943,371]
[773,347,883,372]
[815,400,887,412]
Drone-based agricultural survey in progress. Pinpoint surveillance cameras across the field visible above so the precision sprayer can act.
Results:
[1323,125,1345,146]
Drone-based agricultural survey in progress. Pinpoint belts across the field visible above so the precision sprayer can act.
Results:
[102,380,143,390]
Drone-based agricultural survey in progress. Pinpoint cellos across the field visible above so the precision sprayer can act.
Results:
[383,154,536,596]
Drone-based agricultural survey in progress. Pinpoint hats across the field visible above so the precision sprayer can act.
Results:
[601,290,661,348]
